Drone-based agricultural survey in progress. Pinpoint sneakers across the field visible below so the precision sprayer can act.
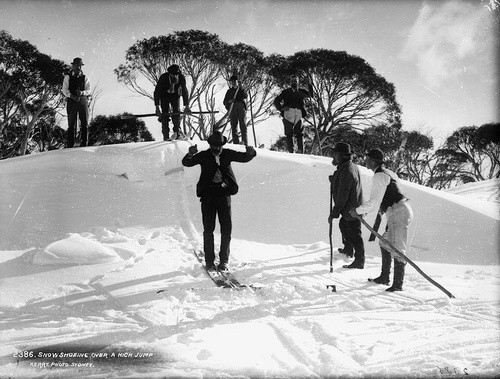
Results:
[206,259,216,269]
[218,260,227,270]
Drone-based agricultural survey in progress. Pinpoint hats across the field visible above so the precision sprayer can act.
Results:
[364,148,387,165]
[167,65,181,75]
[227,76,240,81]
[331,142,352,156]
[71,57,84,66]
[207,131,227,146]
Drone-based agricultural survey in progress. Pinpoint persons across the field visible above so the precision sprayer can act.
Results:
[152,64,193,142]
[328,141,366,270]
[180,130,257,274]
[349,147,415,293]
[222,75,249,147]
[61,56,92,149]
[272,74,315,154]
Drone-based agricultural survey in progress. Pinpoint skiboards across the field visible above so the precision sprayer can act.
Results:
[193,246,246,288]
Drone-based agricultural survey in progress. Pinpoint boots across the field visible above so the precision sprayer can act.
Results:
[173,126,181,140]
[162,128,171,141]
[385,258,406,292]
[368,249,391,284]
